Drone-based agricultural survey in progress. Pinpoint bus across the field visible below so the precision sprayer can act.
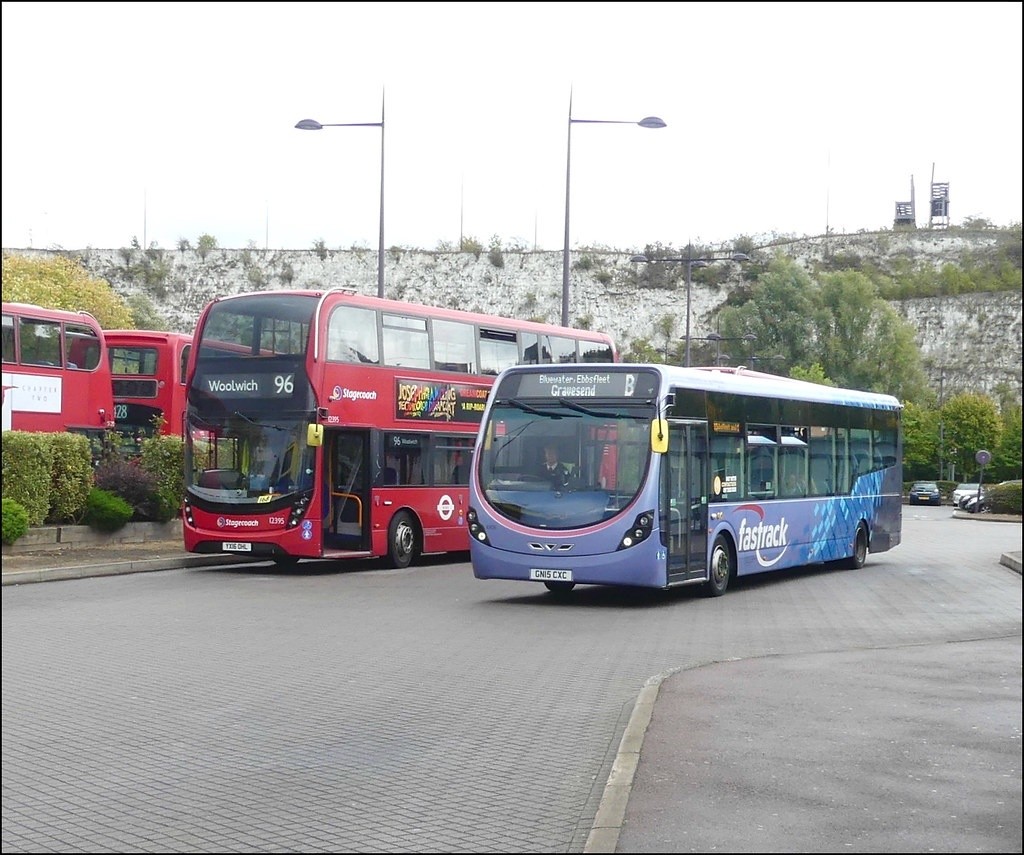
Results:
[2,300,115,486]
[181,290,621,570]
[466,361,904,597]
[68,329,290,483]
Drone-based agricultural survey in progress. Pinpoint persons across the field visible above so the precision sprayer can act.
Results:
[532,443,570,488]
[452,454,469,484]
[786,474,818,496]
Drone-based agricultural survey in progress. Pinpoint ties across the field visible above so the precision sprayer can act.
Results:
[549,466,552,471]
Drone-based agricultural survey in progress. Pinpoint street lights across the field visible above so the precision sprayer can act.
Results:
[292,84,387,299]
[630,238,750,369]
[933,365,947,480]
[560,83,668,329]
[716,340,786,372]
[679,317,759,367]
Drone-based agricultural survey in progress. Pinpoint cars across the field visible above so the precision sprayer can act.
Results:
[909,480,942,506]
[958,479,1023,515]
[952,482,987,507]
